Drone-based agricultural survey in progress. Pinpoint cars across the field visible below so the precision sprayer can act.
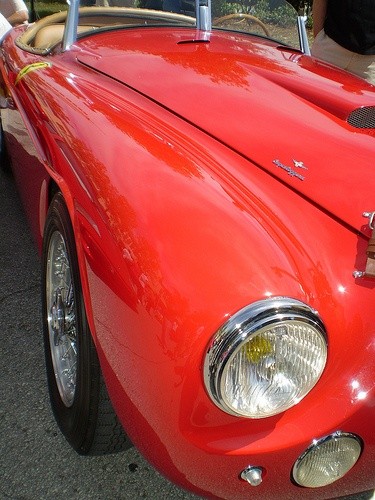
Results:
[0,1,375,500]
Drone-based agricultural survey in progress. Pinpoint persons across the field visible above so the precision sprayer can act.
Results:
[0,0,29,28]
[310,0,375,85]
